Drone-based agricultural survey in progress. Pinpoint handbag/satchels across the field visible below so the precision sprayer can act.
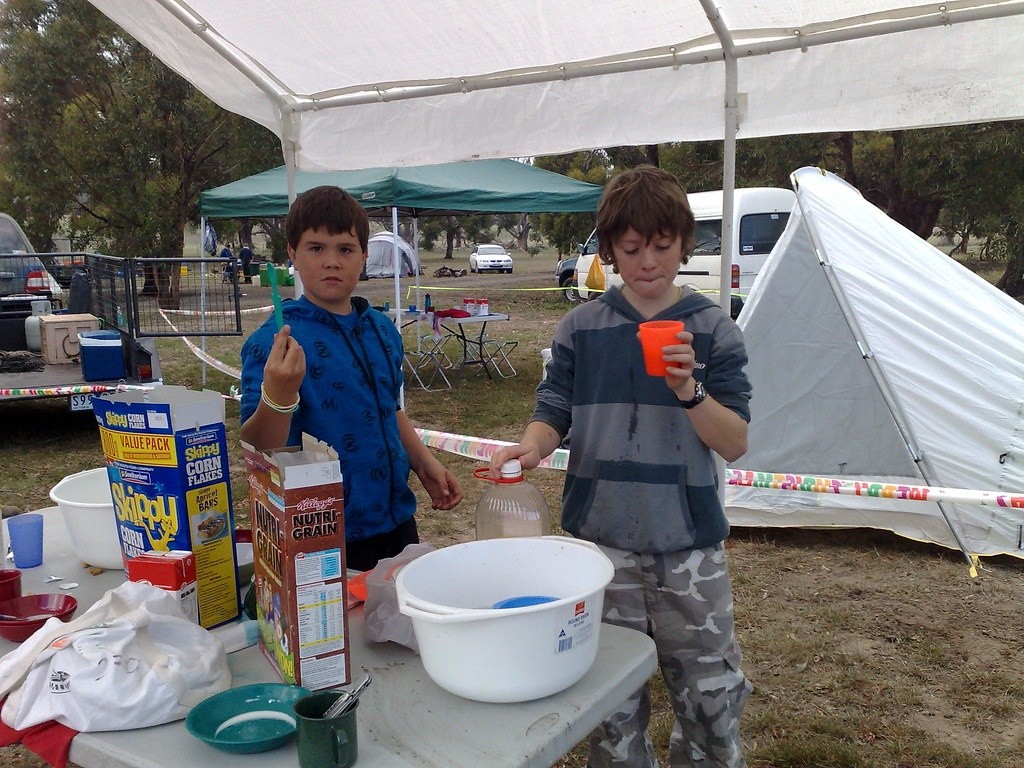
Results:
[0,579,233,732]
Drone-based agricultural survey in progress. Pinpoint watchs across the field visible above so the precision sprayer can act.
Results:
[675,381,707,409]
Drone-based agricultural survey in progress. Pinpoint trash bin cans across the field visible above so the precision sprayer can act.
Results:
[249,262,258,275]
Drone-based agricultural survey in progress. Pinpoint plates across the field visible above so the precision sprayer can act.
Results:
[0,593,78,645]
[229,528,256,588]
[185,682,316,754]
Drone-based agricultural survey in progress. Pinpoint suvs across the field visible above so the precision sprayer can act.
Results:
[0,212,63,319]
[551,257,586,303]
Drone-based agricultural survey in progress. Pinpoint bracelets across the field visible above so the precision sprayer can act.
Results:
[260,382,301,414]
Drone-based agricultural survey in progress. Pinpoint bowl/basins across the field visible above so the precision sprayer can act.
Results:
[396,535,614,704]
[0,568,22,602]
[49,467,126,571]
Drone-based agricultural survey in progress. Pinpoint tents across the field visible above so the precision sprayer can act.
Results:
[724,166,1024,581]
[199,160,605,411]
[366,231,425,278]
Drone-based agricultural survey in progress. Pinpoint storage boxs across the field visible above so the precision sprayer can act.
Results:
[240,433,352,692]
[77,330,125,381]
[127,550,199,625]
[91,385,242,628]
[39,314,99,365]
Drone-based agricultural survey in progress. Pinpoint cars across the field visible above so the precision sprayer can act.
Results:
[468,244,515,274]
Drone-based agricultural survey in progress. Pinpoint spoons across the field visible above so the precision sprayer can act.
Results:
[0,613,52,622]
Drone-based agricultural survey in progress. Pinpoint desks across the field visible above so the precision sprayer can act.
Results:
[0,506,658,768]
[383,309,425,328]
[421,311,509,389]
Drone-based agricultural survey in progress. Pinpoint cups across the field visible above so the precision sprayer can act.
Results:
[7,514,43,568]
[293,689,358,768]
[473,458,552,541]
[638,321,686,378]
[409,305,416,312]
[383,301,389,311]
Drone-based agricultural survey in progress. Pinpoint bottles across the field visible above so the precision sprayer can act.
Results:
[424,293,431,312]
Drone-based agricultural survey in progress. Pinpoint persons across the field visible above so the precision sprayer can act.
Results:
[239,243,253,284]
[220,244,233,284]
[489,164,755,768]
[239,186,464,572]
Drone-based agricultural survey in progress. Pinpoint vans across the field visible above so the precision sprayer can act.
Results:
[573,187,800,319]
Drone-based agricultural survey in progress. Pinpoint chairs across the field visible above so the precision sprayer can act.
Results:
[404,331,519,390]
